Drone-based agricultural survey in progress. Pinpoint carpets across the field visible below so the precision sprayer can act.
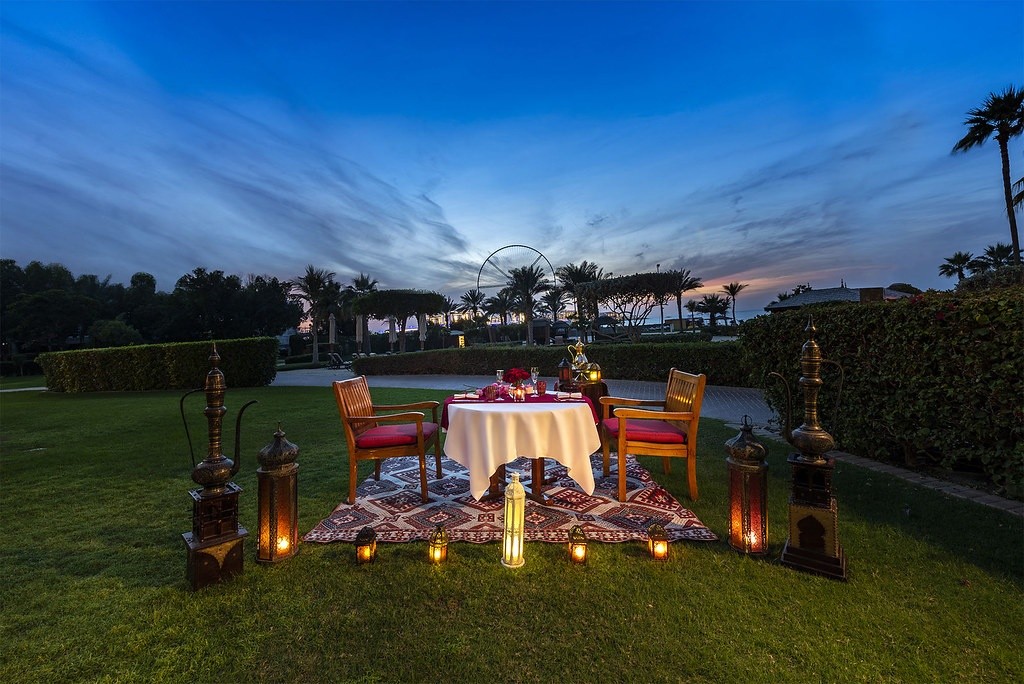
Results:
[301,452,718,543]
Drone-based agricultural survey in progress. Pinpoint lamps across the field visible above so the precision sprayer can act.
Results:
[501,470,526,569]
[558,357,574,392]
[724,413,770,556]
[587,362,602,385]
[429,522,448,564]
[354,525,377,565]
[646,521,669,561]
[256,421,300,564]
[568,524,588,564]
[513,385,526,403]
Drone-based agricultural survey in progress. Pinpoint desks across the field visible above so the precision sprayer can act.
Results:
[439,388,603,502]
[559,382,614,424]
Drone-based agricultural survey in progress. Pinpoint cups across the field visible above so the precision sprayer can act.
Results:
[538,381,546,394]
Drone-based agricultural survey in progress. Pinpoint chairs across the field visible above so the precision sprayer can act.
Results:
[332,373,443,504]
[597,367,708,503]
[328,350,379,371]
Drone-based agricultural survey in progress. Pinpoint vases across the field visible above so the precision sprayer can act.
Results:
[509,382,526,399]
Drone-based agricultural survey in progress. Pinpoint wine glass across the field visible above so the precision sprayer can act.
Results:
[495,370,505,401]
[530,367,540,397]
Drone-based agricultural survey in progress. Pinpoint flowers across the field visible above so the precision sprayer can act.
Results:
[502,367,530,385]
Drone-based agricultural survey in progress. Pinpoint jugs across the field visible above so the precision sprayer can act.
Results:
[179,341,260,496]
[767,313,844,465]
